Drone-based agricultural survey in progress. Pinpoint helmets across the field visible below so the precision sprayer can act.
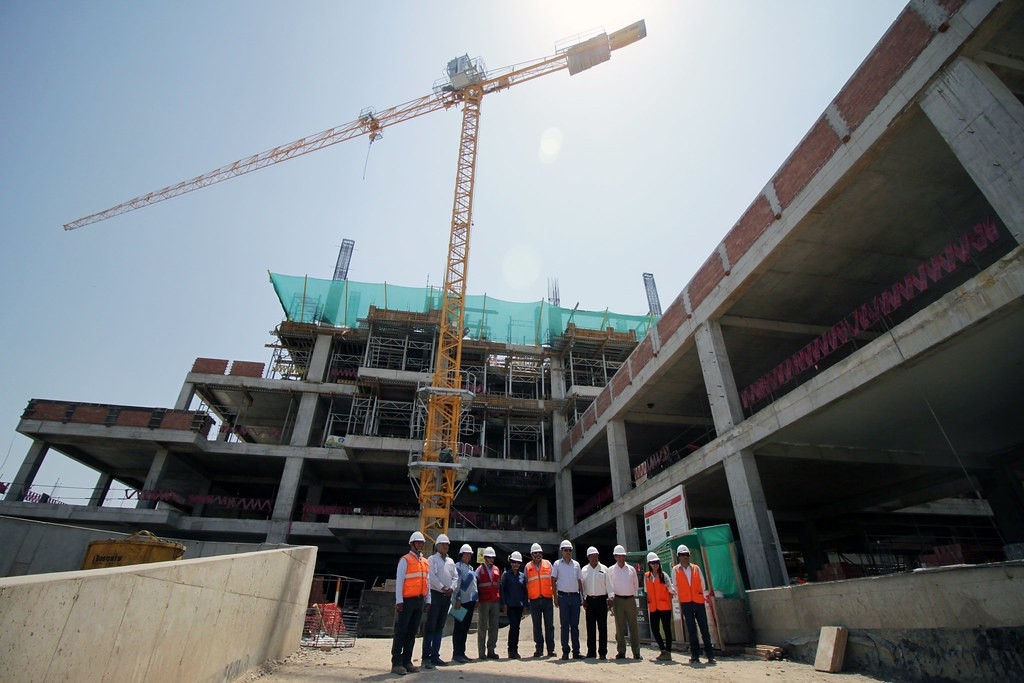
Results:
[587,546,599,557]
[484,547,496,559]
[435,534,450,546]
[647,552,660,564]
[459,544,473,555]
[560,540,573,550]
[409,531,426,545]
[510,551,522,563]
[677,545,690,557]
[613,545,626,556]
[530,543,543,554]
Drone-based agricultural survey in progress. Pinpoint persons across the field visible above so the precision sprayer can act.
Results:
[582,547,612,659]
[452,544,479,663]
[671,544,716,663]
[501,551,529,659]
[524,543,557,658]
[475,547,501,660]
[551,540,586,660]
[390,532,431,675]
[608,545,643,660]
[643,551,675,660]
[420,533,459,670]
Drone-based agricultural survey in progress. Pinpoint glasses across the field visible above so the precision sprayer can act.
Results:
[532,552,542,554]
[485,557,495,559]
[678,553,689,556]
[650,561,660,564]
[563,548,572,552]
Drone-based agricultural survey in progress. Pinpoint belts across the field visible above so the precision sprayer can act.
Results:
[615,594,634,599]
[562,592,578,594]
[586,595,607,599]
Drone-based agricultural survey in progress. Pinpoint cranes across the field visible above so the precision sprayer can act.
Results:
[61,19,642,561]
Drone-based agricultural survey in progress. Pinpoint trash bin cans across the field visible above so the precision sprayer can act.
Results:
[627,596,651,639]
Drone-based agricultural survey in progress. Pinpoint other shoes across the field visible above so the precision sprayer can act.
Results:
[689,656,699,663]
[392,652,643,675]
[709,656,717,664]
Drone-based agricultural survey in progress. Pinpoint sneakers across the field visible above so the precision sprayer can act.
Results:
[657,650,671,661]
